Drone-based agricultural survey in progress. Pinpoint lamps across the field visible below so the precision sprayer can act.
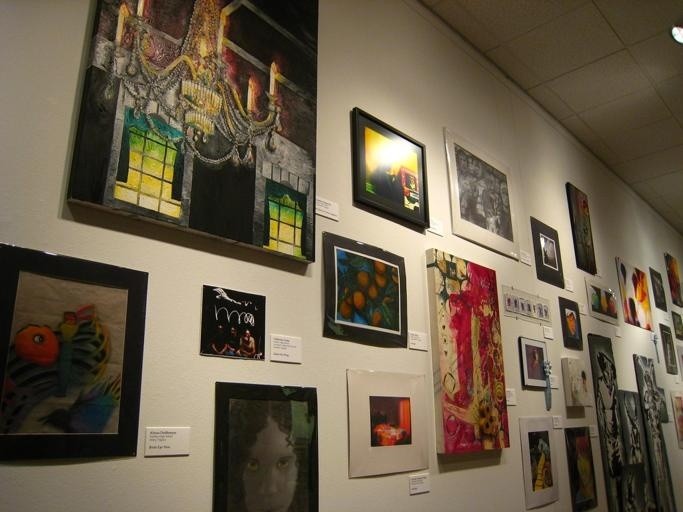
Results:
[105,1,285,174]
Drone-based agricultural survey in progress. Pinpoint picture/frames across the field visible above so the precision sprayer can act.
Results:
[650,268,667,311]
[1,243,150,470]
[659,308,682,375]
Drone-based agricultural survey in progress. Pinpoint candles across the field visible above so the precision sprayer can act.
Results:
[268,68,276,96]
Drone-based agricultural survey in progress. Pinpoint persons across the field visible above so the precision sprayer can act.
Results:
[565,308,580,339]
[541,235,558,271]
[525,344,545,380]
[207,323,256,358]
[228,399,313,512]
[594,350,675,512]
[568,367,593,403]
